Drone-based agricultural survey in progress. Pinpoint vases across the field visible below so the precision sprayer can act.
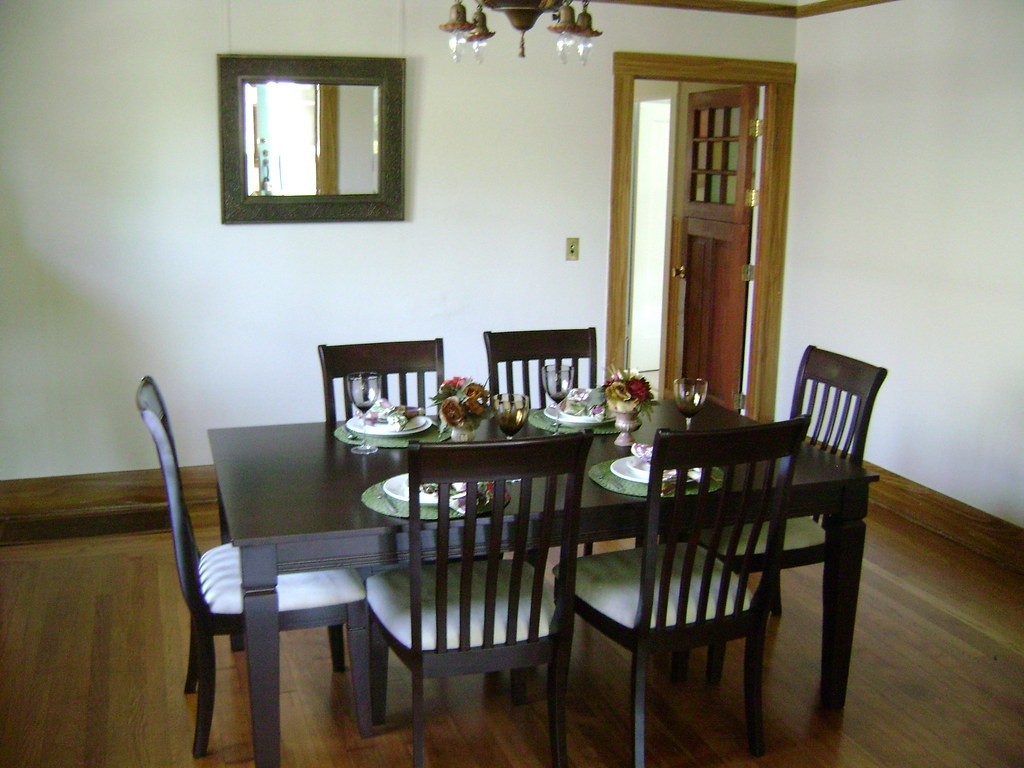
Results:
[613,409,646,448]
[450,416,491,442]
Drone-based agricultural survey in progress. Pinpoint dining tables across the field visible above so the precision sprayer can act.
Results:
[207,397,880,768]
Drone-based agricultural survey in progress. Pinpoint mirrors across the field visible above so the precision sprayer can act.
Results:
[219,52,405,223]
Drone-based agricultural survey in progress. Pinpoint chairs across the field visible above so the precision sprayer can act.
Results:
[318,327,812,768]
[700,346,888,688]
[133,376,375,759]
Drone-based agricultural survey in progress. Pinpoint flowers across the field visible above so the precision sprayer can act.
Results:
[430,377,496,438]
[596,362,656,424]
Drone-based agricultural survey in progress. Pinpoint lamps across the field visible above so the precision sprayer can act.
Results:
[439,0,602,59]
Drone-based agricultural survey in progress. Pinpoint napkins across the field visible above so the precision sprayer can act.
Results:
[554,386,608,417]
[422,481,512,515]
[631,443,701,482]
[356,396,425,429]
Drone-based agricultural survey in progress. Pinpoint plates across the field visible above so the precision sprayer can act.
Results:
[544,401,624,427]
[608,455,702,484]
[382,472,480,504]
[347,409,434,437]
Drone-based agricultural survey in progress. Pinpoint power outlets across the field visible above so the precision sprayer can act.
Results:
[565,237,580,261]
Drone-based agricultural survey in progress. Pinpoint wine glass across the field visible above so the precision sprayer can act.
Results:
[345,371,383,455]
[541,365,575,434]
[673,377,709,432]
[491,393,530,442]
